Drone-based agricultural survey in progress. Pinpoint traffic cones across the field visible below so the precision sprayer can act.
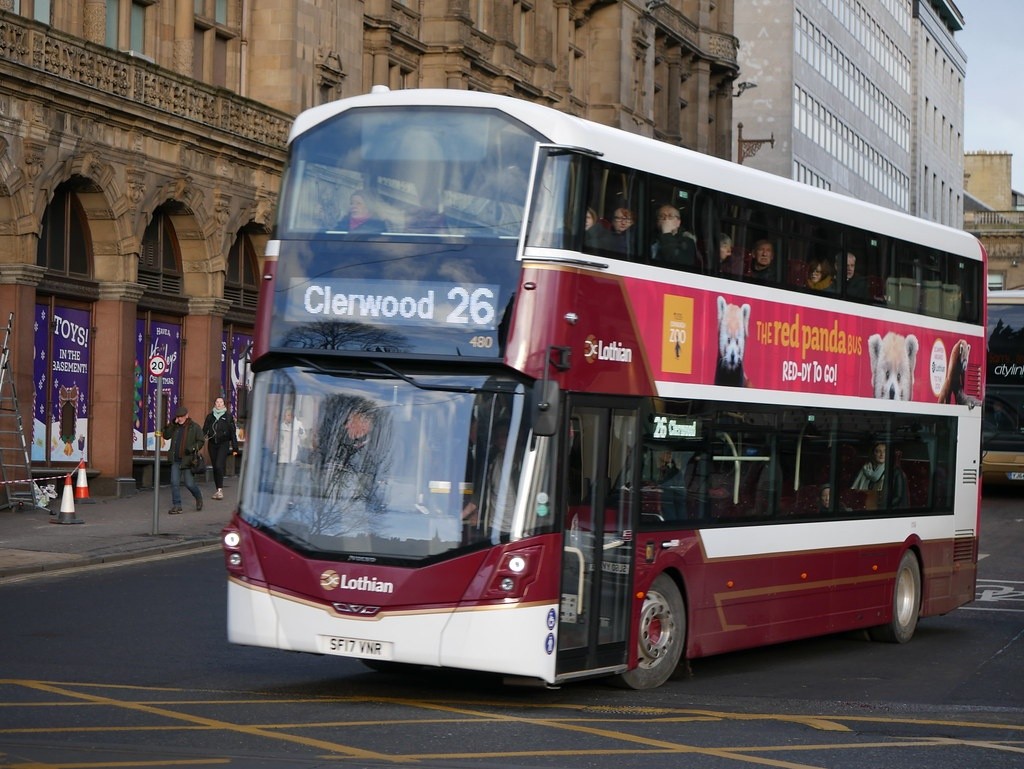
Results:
[49,473,85,524]
[74,458,97,504]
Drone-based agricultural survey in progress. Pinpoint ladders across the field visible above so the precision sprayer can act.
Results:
[0,311,37,510]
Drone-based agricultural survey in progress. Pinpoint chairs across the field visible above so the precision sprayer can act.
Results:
[885,276,961,321]
[625,445,944,522]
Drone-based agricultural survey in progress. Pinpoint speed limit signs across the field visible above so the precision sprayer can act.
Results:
[149,355,166,377]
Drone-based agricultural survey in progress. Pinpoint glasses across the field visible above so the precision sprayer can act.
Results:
[656,213,681,220]
[613,216,633,223]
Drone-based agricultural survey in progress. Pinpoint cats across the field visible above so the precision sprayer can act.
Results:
[936,338,970,404]
[714,296,754,389]
[867,331,919,402]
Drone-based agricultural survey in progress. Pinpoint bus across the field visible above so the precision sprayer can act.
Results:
[979,288,1024,495]
[222,87,993,695]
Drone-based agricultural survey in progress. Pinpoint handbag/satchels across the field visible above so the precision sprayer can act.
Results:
[190,443,207,476]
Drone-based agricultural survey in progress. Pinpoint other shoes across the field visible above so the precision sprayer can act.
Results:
[218,488,223,499]
[196,499,203,510]
[212,491,217,499]
[168,506,182,515]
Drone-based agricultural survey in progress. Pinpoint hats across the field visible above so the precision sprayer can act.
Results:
[174,407,188,416]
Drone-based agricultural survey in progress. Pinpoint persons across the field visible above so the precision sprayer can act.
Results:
[803,257,838,299]
[846,252,867,300]
[646,206,697,268]
[274,407,306,466]
[415,403,532,541]
[619,445,729,522]
[333,166,525,236]
[162,408,205,514]
[201,397,238,499]
[747,238,779,289]
[817,435,909,516]
[715,233,738,280]
[585,206,635,257]
